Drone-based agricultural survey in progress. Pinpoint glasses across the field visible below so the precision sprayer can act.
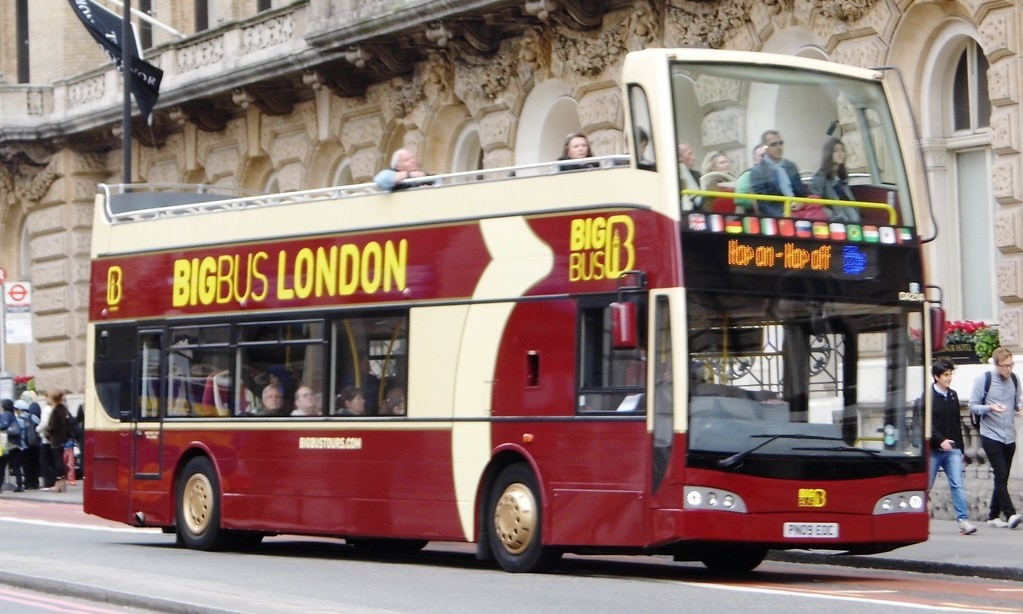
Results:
[997,362,1014,369]
[769,141,783,146]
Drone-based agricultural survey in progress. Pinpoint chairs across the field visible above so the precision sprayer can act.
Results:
[141,349,195,417]
[201,370,254,416]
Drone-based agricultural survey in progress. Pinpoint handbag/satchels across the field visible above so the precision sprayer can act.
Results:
[7,412,20,438]
[65,408,83,438]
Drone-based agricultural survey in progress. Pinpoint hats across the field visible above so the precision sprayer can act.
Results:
[13,400,31,410]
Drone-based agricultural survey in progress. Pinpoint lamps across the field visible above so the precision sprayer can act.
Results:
[826,120,839,135]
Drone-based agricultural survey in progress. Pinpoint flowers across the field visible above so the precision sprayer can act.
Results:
[908,320,990,346]
[12,376,33,389]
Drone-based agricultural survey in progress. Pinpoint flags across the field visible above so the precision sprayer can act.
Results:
[70,0,164,124]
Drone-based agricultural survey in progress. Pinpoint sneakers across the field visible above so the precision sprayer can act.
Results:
[1008,513,1022,528]
[959,521,977,535]
[988,518,1007,527]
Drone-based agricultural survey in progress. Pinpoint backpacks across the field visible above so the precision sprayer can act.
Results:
[970,372,1018,432]
[19,413,41,447]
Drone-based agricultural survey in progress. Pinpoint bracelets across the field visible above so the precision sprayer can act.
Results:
[406,171,410,177]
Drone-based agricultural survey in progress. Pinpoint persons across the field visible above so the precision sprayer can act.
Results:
[809,136,862,222]
[677,143,702,186]
[733,144,765,213]
[624,341,669,396]
[242,358,293,411]
[967,347,1023,528]
[612,128,648,165]
[291,385,322,416]
[751,129,827,221]
[373,149,441,192]
[557,133,599,171]
[919,360,977,535]
[0,388,84,494]
[701,151,730,174]
[253,384,288,416]
[336,349,405,415]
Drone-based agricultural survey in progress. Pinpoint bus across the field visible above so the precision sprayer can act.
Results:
[81,47,948,572]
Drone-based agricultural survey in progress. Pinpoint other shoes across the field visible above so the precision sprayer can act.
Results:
[15,479,77,493]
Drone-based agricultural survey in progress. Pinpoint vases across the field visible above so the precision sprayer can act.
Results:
[15,387,26,400]
[913,342,979,365]
